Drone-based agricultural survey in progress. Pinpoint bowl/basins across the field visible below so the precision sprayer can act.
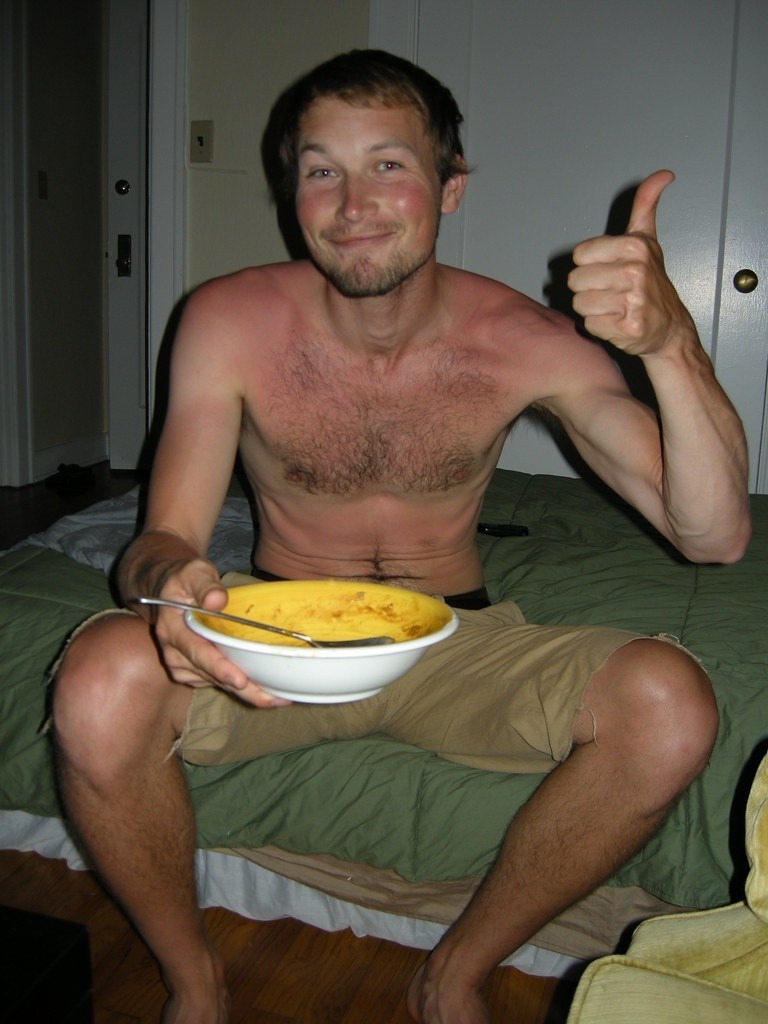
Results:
[185,579,460,704]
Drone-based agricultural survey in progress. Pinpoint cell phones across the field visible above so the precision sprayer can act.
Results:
[478,523,529,537]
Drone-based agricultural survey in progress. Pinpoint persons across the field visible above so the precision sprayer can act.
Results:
[44,49,751,1024]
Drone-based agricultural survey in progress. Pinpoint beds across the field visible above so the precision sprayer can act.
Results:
[0,468,768,973]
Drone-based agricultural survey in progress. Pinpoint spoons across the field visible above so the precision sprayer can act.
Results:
[136,597,396,648]
